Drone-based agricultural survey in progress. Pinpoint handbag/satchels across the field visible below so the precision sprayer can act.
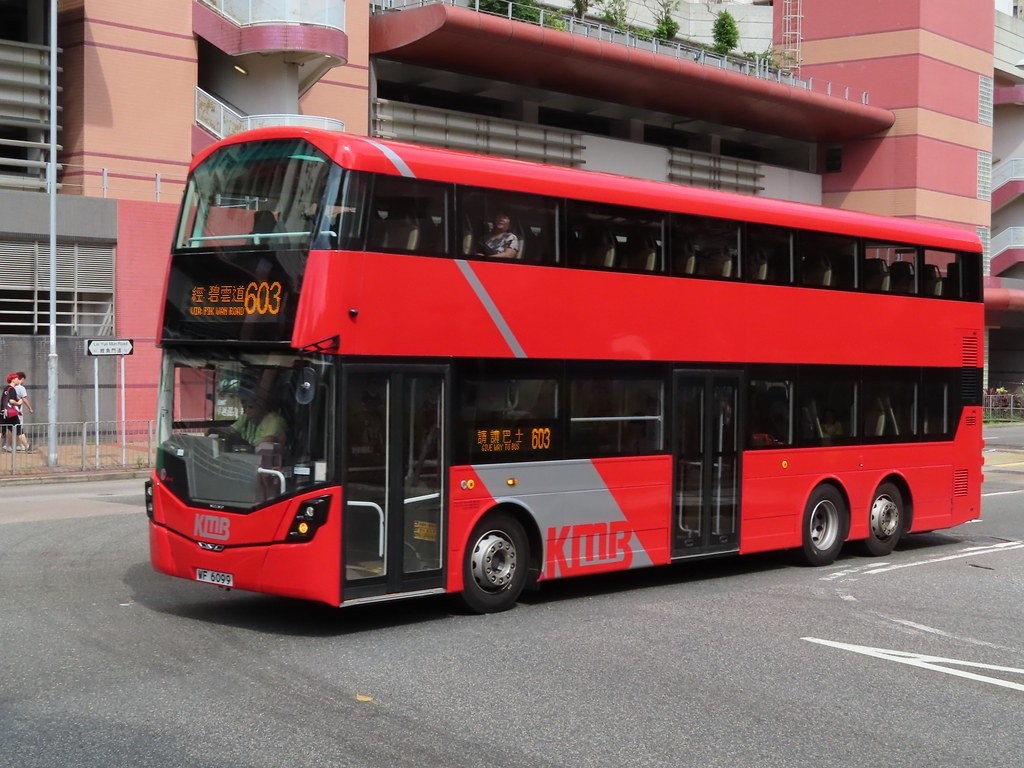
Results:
[4,408,18,418]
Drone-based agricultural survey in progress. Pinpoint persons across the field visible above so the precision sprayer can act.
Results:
[2,372,34,452]
[411,427,459,490]
[0,374,38,452]
[818,409,843,438]
[223,385,287,448]
[268,206,331,249]
[475,210,519,258]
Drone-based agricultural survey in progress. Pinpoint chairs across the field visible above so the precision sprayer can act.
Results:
[277,381,934,463]
[245,208,946,298]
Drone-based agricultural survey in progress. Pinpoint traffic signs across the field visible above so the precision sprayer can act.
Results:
[83,340,134,356]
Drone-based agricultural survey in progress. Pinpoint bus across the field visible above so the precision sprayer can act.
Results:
[146,126,986,617]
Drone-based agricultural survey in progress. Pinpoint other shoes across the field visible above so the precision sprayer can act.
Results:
[3,445,13,452]
[27,448,38,454]
[17,445,26,451]
